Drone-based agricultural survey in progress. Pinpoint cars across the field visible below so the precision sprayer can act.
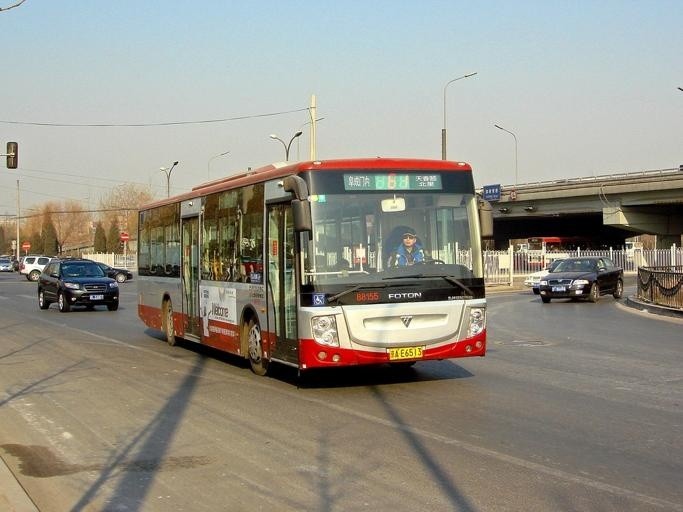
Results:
[537,255,625,304]
[522,257,562,295]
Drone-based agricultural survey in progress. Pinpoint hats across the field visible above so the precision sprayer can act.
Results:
[402,228,417,237]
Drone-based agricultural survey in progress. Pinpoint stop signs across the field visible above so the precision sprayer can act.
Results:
[22,241,30,251]
[119,231,130,242]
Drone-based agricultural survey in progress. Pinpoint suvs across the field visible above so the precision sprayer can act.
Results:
[0,254,133,314]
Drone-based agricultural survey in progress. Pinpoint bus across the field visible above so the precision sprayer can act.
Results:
[624,240,643,255]
[525,235,627,269]
[133,156,489,386]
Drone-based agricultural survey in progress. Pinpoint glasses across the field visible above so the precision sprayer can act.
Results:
[402,235,416,239]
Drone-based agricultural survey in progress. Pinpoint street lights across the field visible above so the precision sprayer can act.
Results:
[158,160,179,199]
[268,130,303,163]
[296,117,326,162]
[206,150,230,181]
[440,70,478,163]
[676,86,682,93]
[491,124,518,186]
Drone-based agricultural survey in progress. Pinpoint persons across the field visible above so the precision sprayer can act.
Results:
[387,229,427,268]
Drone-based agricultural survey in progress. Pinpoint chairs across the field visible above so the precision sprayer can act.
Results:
[386,225,422,268]
